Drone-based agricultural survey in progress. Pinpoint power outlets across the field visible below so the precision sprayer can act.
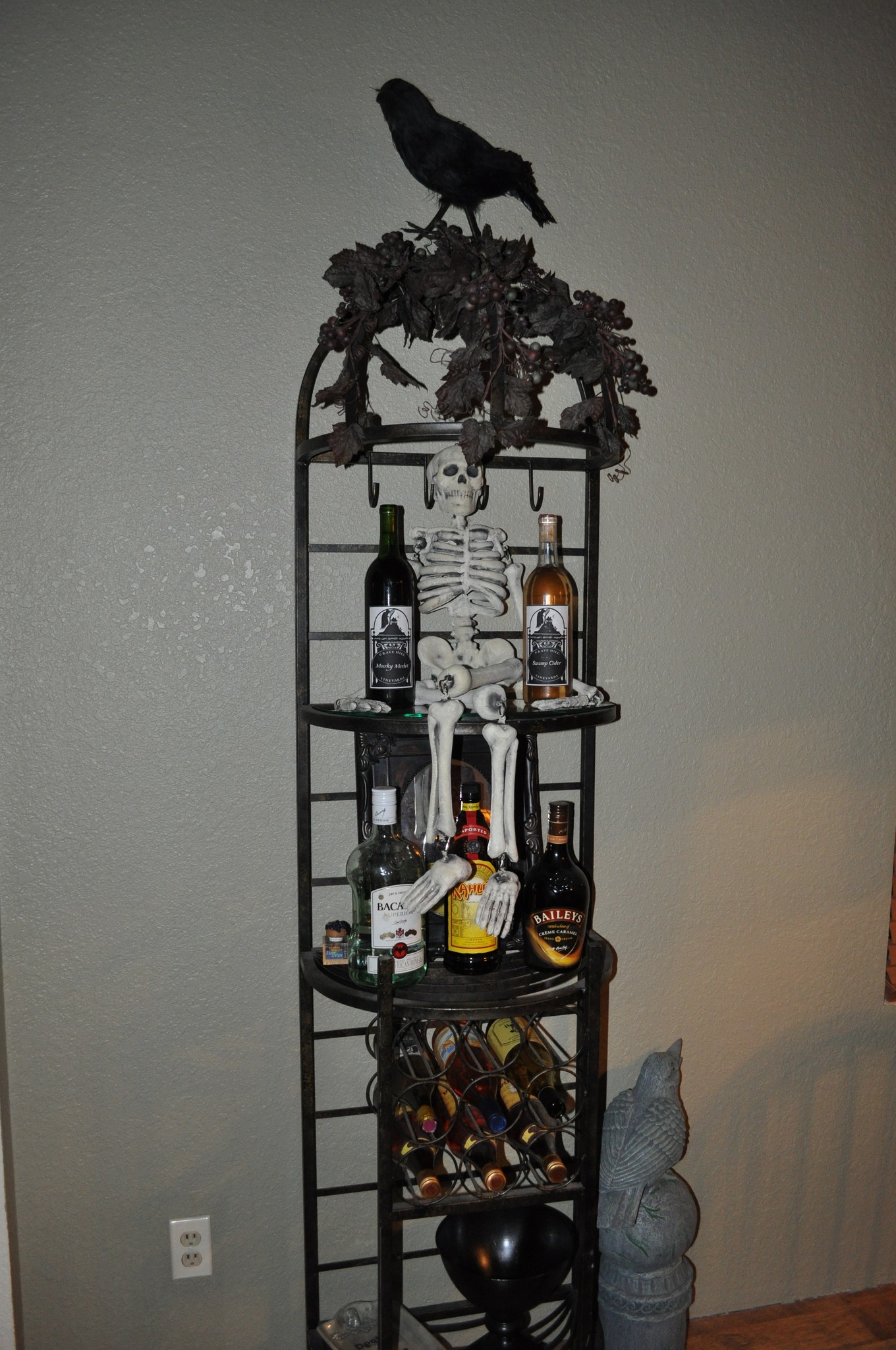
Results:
[167,1213,217,1284]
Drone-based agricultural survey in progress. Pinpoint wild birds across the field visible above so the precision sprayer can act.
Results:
[594,1036,690,1230]
[365,75,559,250]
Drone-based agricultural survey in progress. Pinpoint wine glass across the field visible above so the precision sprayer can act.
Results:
[435,1202,574,1350]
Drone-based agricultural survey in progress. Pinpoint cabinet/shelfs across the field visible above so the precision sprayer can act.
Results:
[283,74,662,1350]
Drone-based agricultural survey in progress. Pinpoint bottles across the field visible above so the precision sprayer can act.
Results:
[522,800,590,974]
[346,786,427,988]
[523,514,579,708]
[422,781,499,976]
[373,1016,568,1200]
[364,504,421,710]
